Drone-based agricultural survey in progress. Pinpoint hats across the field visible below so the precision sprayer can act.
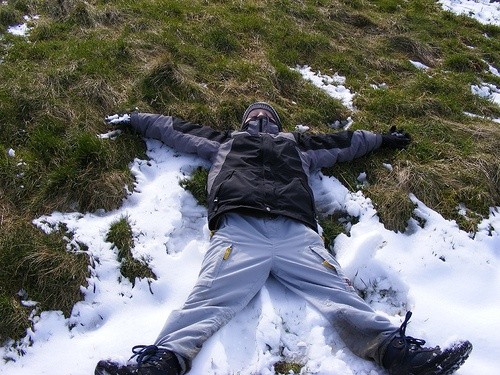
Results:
[241,102,281,129]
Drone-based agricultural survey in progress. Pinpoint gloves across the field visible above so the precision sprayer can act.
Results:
[382,135,410,148]
[106,114,130,125]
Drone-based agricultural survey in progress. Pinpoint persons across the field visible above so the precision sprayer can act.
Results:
[93,100,473,375]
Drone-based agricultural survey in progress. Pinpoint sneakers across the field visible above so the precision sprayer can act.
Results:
[94,345,180,375]
[383,338,475,375]
